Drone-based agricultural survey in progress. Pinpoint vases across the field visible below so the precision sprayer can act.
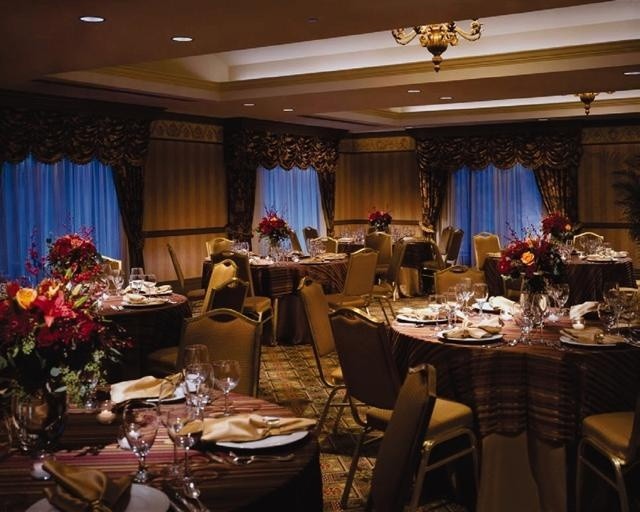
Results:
[0,377,67,459]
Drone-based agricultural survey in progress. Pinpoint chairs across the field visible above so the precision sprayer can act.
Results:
[101,255,122,294]
[167,227,639,510]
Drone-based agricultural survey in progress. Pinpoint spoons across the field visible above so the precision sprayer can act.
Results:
[182,481,209,511]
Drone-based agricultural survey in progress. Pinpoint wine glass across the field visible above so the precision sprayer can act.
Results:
[121,343,239,498]
[231,228,414,266]
[101,266,173,308]
[562,233,628,263]
[399,277,640,351]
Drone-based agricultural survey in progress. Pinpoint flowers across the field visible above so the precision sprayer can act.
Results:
[367,206,394,229]
[540,209,584,242]
[254,200,294,243]
[0,227,134,410]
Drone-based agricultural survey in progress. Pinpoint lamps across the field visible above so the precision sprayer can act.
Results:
[567,89,616,115]
[392,17,484,74]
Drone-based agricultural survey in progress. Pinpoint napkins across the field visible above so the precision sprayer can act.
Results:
[43,461,133,512]
[109,369,186,413]
[123,281,171,305]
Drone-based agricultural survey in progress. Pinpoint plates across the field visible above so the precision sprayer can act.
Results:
[24,482,169,511]
[216,416,310,448]
